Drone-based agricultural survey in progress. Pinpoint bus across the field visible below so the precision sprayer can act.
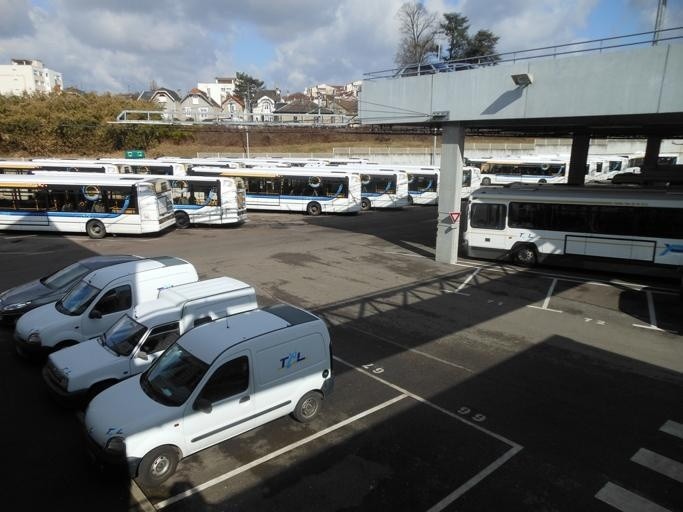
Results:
[151,175,248,230]
[0,172,177,239]
[461,184,681,280]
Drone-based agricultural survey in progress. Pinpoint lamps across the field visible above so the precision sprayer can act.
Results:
[510,73,531,87]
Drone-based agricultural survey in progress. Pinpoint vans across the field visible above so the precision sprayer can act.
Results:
[11,256,201,367]
[85,301,335,490]
[42,275,260,416]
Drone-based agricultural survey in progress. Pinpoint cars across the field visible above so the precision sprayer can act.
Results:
[0,255,148,330]
